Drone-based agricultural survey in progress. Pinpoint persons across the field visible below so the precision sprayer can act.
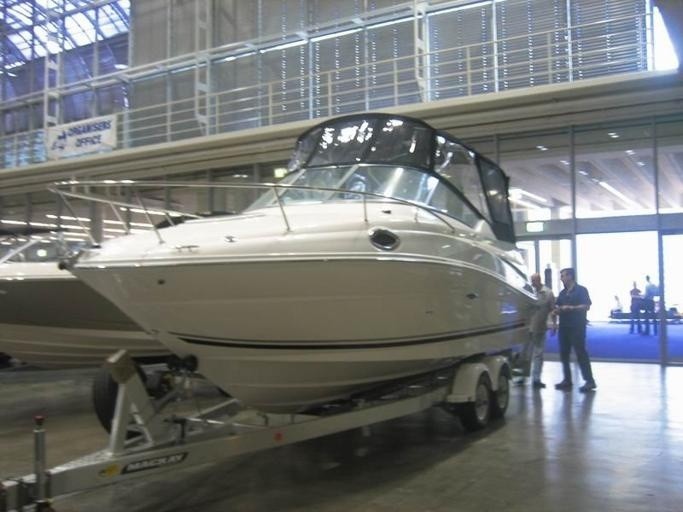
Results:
[641,274,660,335]
[608,294,624,318]
[551,267,598,392]
[512,272,558,388]
[629,281,643,334]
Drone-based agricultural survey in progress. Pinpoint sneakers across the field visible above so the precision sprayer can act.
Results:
[513,379,597,392]
